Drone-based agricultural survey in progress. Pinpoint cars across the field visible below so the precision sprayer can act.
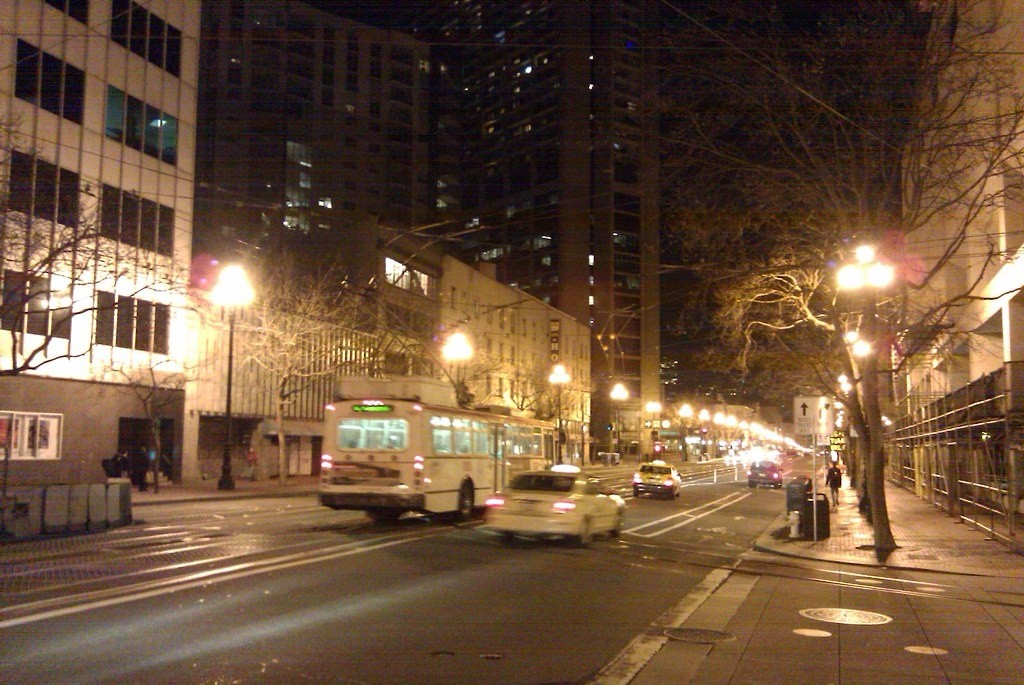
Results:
[483,471,626,548]
[633,460,683,500]
[748,460,784,490]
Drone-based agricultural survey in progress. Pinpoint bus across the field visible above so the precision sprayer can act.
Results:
[318,396,567,523]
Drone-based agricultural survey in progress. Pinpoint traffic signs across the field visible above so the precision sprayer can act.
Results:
[794,396,834,435]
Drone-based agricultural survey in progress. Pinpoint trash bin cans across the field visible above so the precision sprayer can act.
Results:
[802,492,831,541]
[786,477,813,519]
[602,453,620,467]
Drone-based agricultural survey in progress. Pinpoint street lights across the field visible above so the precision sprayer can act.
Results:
[212,265,253,491]
[549,364,569,465]
[611,384,628,453]
[444,333,472,382]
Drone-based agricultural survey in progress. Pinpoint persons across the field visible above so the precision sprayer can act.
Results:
[134,446,149,491]
[246,448,256,481]
[825,461,842,506]
[114,447,130,478]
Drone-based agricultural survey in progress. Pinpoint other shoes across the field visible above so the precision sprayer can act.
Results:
[833,503,835,506]
[836,502,839,505]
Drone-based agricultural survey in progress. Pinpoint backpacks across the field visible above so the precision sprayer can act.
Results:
[102,459,118,477]
